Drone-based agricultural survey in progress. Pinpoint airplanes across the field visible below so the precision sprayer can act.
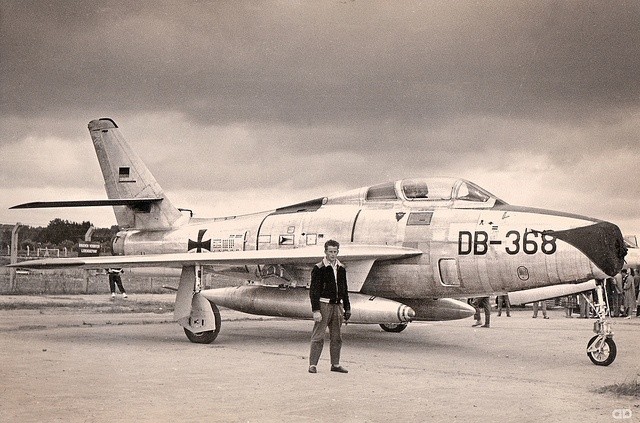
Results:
[6,118,628,366]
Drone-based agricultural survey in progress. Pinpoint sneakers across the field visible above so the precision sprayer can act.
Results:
[481,324,489,328]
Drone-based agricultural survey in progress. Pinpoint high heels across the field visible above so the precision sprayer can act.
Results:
[624,316,631,319]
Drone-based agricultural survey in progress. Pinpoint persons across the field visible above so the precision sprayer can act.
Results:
[531,301,549,319]
[308,239,351,374]
[496,296,512,318]
[624,267,636,318]
[633,269,640,315]
[612,268,628,316]
[562,293,577,318]
[577,291,589,318]
[471,298,491,328]
[104,267,129,298]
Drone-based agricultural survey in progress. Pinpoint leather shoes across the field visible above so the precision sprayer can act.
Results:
[309,366,316,373]
[331,365,348,373]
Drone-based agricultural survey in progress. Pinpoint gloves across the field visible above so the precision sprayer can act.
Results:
[343,309,351,320]
